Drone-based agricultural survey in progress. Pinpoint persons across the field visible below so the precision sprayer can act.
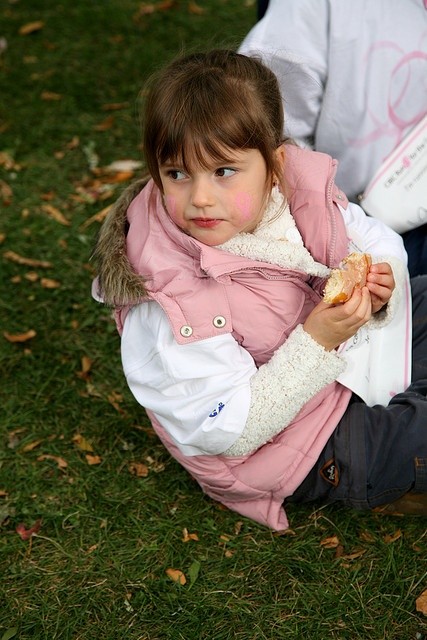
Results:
[238,1,425,234]
[90,50,426,530]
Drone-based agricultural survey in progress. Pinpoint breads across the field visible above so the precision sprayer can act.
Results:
[321,252,372,304]
